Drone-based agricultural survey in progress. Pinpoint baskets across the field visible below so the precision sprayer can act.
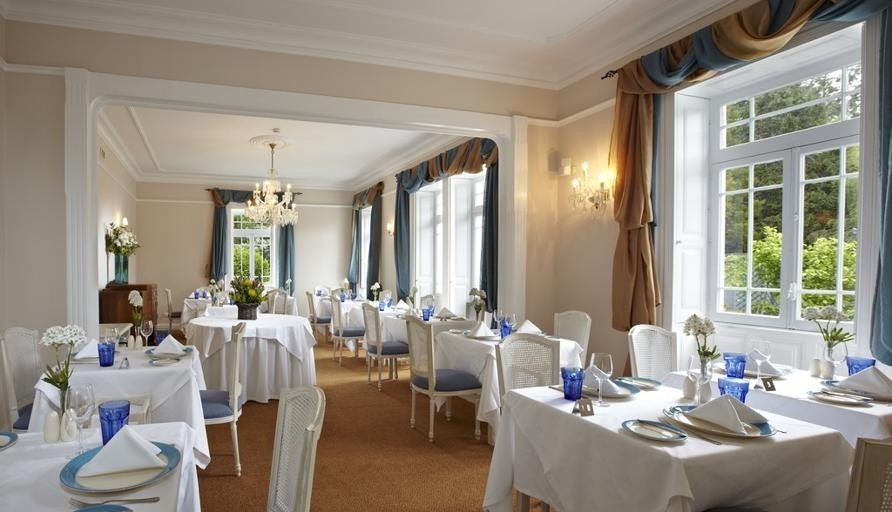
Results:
[235,303,259,320]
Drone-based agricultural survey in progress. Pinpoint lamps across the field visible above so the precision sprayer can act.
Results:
[243,143,300,227]
[569,160,617,216]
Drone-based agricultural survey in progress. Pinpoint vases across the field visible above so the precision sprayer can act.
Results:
[115,253,129,285]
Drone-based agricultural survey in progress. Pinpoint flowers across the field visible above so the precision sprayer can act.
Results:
[105,221,142,257]
[228,271,269,303]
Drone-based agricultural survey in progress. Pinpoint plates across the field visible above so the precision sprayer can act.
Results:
[73,503,134,512]
[807,389,872,405]
[820,379,874,397]
[0,432,19,450]
[663,404,776,437]
[145,348,193,357]
[591,379,640,398]
[464,329,501,339]
[65,351,118,361]
[149,358,181,365]
[620,376,663,388]
[622,418,688,443]
[714,362,778,377]
[60,441,182,493]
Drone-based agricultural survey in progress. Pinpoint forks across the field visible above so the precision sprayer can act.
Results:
[659,415,722,447]
[69,496,161,507]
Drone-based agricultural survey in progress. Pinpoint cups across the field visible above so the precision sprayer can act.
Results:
[428,306,435,316]
[723,352,747,379]
[97,342,115,367]
[387,298,393,307]
[560,366,584,400]
[718,377,749,403]
[194,291,199,299]
[203,289,208,297]
[350,293,357,300]
[846,356,876,376]
[379,302,385,310]
[339,294,345,302]
[98,400,130,445]
[422,309,430,321]
[499,317,510,326]
[501,326,511,338]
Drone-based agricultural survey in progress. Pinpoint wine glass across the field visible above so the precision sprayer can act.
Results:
[589,351,613,406]
[140,320,153,346]
[823,340,848,378]
[494,309,503,328]
[686,355,713,403]
[105,327,119,346]
[65,384,96,456]
[505,312,521,331]
